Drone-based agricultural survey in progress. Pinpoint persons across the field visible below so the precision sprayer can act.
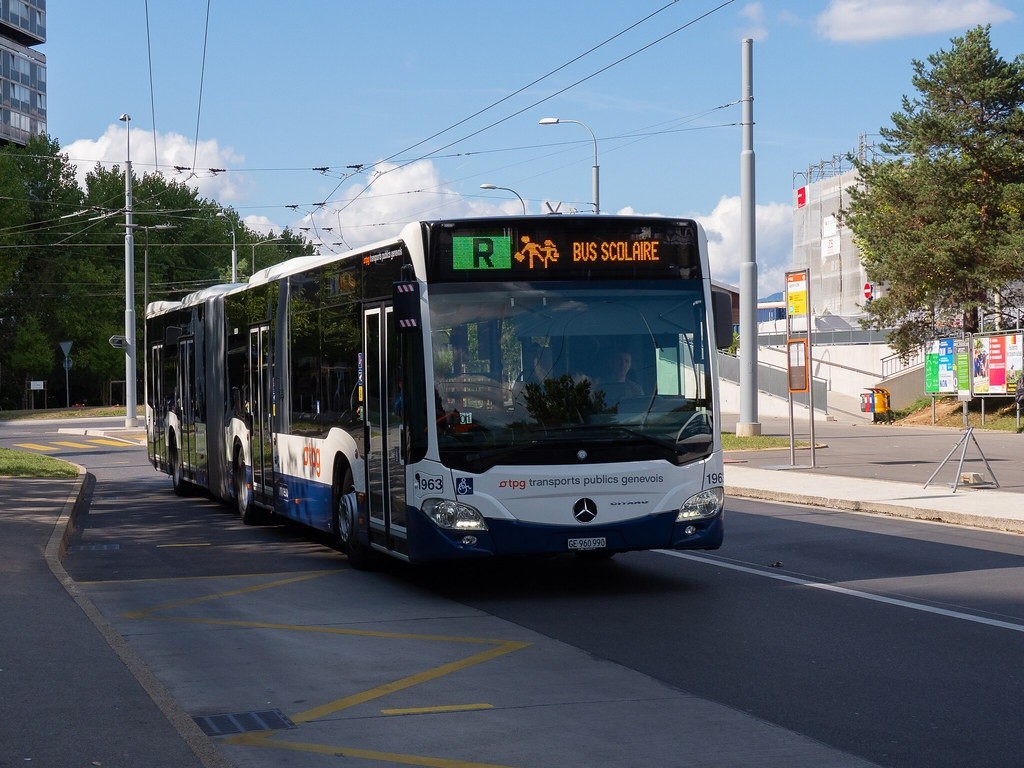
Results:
[1010,365,1015,380]
[598,349,645,400]
[512,347,553,410]
[974,349,986,377]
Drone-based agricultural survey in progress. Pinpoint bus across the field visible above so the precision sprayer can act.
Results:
[145,212,733,570]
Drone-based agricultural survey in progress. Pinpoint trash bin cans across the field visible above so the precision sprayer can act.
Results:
[860,388,892,426]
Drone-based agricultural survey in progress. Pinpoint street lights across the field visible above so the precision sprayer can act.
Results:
[539,117,601,215]
[216,213,238,285]
[480,184,526,215]
[114,222,177,313]
[251,238,286,276]
[119,112,139,427]
